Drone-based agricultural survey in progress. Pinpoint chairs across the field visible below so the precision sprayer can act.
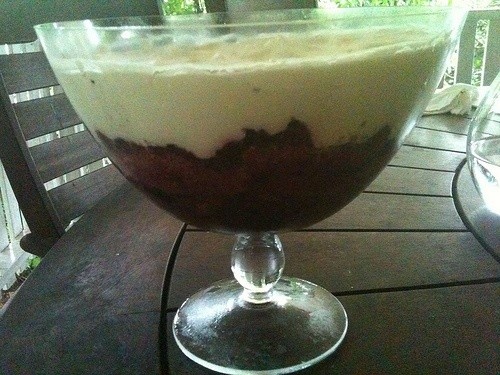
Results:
[0,1,184,261]
[453,8,500,86]
[203,0,322,37]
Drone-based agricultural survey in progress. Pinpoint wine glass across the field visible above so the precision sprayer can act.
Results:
[33,7,462,375]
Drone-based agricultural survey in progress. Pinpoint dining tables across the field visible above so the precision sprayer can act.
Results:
[2,86,499,375]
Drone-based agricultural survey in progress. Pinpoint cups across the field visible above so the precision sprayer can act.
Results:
[466,73,498,216]
